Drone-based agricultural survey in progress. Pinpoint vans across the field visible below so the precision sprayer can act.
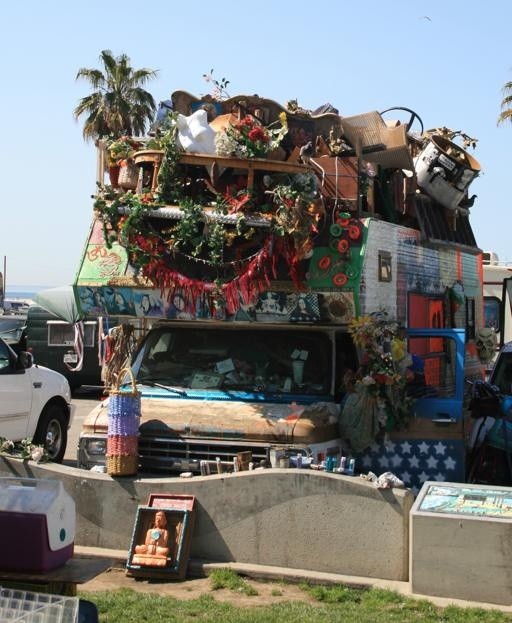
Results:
[482,257,511,376]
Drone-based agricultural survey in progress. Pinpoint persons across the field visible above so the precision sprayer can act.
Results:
[131,508,174,568]
[80,286,319,322]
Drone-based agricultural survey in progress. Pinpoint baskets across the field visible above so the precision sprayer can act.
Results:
[106,368,141,476]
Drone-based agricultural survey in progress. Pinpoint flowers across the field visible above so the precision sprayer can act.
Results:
[0,435,52,464]
[107,132,142,169]
[214,114,271,159]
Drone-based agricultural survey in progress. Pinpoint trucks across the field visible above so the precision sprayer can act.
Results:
[79,205,484,495]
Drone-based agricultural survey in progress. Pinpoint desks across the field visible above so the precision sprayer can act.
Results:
[1,557,117,599]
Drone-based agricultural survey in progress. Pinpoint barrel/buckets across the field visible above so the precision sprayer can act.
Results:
[413,135,482,212]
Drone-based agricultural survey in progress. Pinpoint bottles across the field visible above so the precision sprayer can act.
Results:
[324,455,355,475]
[297,454,302,469]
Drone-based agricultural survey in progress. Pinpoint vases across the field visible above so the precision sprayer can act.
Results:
[119,164,141,190]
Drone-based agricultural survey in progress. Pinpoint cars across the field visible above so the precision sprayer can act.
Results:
[486,341,512,483]
[0,296,113,464]
